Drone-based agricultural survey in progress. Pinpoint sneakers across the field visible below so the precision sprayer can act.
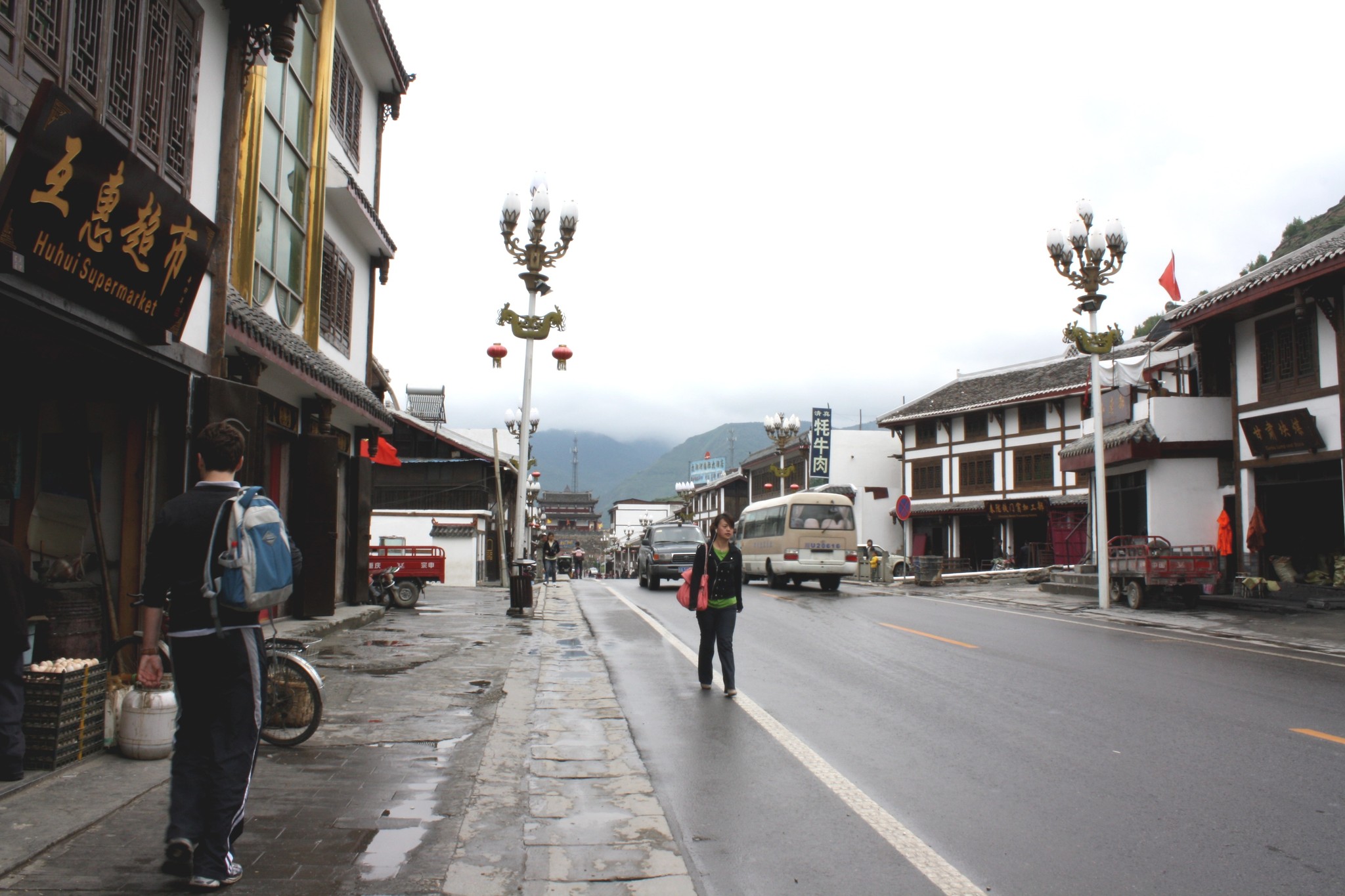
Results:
[189,863,243,887]
[165,837,194,875]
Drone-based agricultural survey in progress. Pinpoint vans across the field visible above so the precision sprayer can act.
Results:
[590,568,598,575]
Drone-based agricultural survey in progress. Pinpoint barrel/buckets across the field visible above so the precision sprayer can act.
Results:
[22,625,35,665]
[118,671,178,761]
[38,581,101,661]
[913,555,943,586]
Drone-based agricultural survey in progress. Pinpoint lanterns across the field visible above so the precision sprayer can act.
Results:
[532,471,540,482]
[610,547,636,556]
[529,521,541,531]
[764,483,773,492]
[552,345,573,371]
[790,484,799,493]
[487,343,508,368]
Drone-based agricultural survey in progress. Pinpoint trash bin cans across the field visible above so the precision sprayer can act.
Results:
[510,558,535,614]
[912,555,943,586]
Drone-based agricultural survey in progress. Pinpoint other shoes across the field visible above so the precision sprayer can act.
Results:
[543,582,548,586]
[727,688,737,696]
[553,582,556,586]
[869,580,872,582]
[700,682,711,689]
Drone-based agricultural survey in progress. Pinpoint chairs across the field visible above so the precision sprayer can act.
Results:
[754,518,853,536]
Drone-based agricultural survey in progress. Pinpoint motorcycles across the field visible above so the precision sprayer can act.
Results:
[557,556,572,579]
[369,545,445,609]
[1106,534,1218,610]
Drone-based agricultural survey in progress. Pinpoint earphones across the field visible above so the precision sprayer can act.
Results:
[716,529,718,533]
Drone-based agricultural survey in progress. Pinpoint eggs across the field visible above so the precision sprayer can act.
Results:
[30,657,99,673]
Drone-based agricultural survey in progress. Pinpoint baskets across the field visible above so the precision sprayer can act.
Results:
[263,635,321,680]
[268,672,326,728]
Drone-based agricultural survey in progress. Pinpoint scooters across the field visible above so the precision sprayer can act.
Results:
[361,566,399,612]
[991,552,1016,571]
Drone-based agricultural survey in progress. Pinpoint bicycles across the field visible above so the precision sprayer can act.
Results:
[106,593,326,748]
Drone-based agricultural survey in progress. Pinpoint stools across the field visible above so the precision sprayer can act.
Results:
[1242,577,1267,599]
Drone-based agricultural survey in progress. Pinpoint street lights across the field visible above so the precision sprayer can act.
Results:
[496,169,581,617]
[764,412,800,497]
[600,534,609,575]
[624,522,635,579]
[1047,194,1130,608]
[609,529,618,579]
[675,481,695,520]
[525,474,546,561]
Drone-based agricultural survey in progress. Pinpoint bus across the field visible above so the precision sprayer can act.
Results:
[730,492,859,592]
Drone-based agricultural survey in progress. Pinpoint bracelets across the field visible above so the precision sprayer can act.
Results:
[140,648,159,655]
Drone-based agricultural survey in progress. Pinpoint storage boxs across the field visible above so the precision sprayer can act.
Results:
[19,654,108,772]
[912,555,943,586]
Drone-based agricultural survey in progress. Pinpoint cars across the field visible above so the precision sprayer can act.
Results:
[857,544,912,578]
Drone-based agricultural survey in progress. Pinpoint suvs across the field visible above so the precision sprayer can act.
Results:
[637,521,710,591]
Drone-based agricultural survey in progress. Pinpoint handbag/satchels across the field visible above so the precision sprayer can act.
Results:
[677,543,709,611]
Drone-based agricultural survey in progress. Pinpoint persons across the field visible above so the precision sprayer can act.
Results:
[992,539,1028,568]
[922,548,949,559]
[531,532,560,587]
[0,539,30,782]
[676,513,743,696]
[595,568,638,579]
[137,421,293,887]
[560,541,585,579]
[584,569,591,578]
[1147,379,1171,399]
[506,547,514,561]
[863,539,879,583]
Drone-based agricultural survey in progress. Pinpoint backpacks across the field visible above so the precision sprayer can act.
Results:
[201,485,294,612]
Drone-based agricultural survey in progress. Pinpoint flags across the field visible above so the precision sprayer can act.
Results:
[1159,250,1181,301]
[360,436,401,467]
